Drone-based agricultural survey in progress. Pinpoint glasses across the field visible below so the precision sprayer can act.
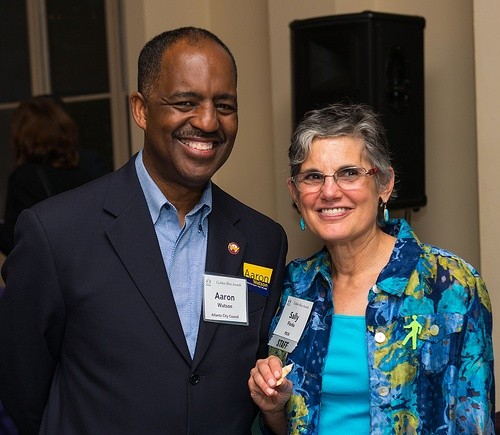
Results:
[292,167,379,194]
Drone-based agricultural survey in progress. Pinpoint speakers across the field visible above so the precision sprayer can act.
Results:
[289,10,427,212]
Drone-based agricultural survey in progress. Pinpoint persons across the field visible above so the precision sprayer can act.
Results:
[0,28,288,435]
[0,94,106,258]
[247,106,495,435]
